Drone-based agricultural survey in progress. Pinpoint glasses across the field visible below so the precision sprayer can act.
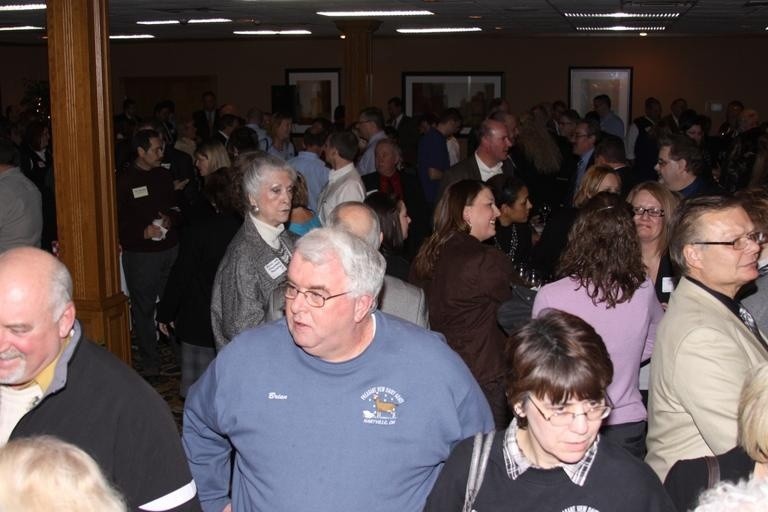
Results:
[528,394,614,427]
[278,280,351,308]
[692,230,763,250]
[633,206,665,218]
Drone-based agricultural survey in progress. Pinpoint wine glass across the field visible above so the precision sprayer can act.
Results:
[515,262,541,290]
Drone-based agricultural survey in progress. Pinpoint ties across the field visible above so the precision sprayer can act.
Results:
[738,302,768,354]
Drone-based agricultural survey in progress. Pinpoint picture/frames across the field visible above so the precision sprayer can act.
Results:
[284,68,341,137]
[401,71,505,118]
[567,65,633,132]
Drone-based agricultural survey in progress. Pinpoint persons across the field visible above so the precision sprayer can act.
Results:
[0,245,202,511]
[0,433,129,511]
[640,193,768,483]
[528,191,665,461]
[181,226,497,511]
[317,94,628,283]
[112,89,333,397]
[626,97,767,199]
[412,177,537,432]
[690,360,768,512]
[0,103,58,257]
[423,306,678,512]
